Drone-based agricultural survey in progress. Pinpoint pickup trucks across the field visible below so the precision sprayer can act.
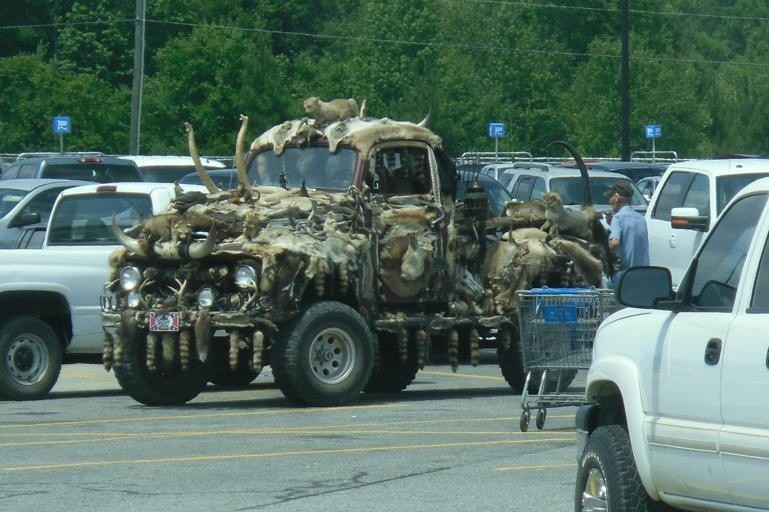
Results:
[1,182,223,400]
[600,158,769,289]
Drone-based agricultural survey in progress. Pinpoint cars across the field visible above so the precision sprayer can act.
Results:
[458,160,671,232]
[0,148,239,250]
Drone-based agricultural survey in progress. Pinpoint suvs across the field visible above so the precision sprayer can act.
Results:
[575,177,769,511]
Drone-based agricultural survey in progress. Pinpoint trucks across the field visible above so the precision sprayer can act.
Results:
[100,98,623,407]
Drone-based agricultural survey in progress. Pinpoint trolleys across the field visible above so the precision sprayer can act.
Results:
[516,287,616,431]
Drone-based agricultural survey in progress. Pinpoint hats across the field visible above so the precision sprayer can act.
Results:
[602,182,635,197]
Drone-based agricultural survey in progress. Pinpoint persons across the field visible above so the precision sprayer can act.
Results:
[599,181,650,288]
[252,148,352,190]
